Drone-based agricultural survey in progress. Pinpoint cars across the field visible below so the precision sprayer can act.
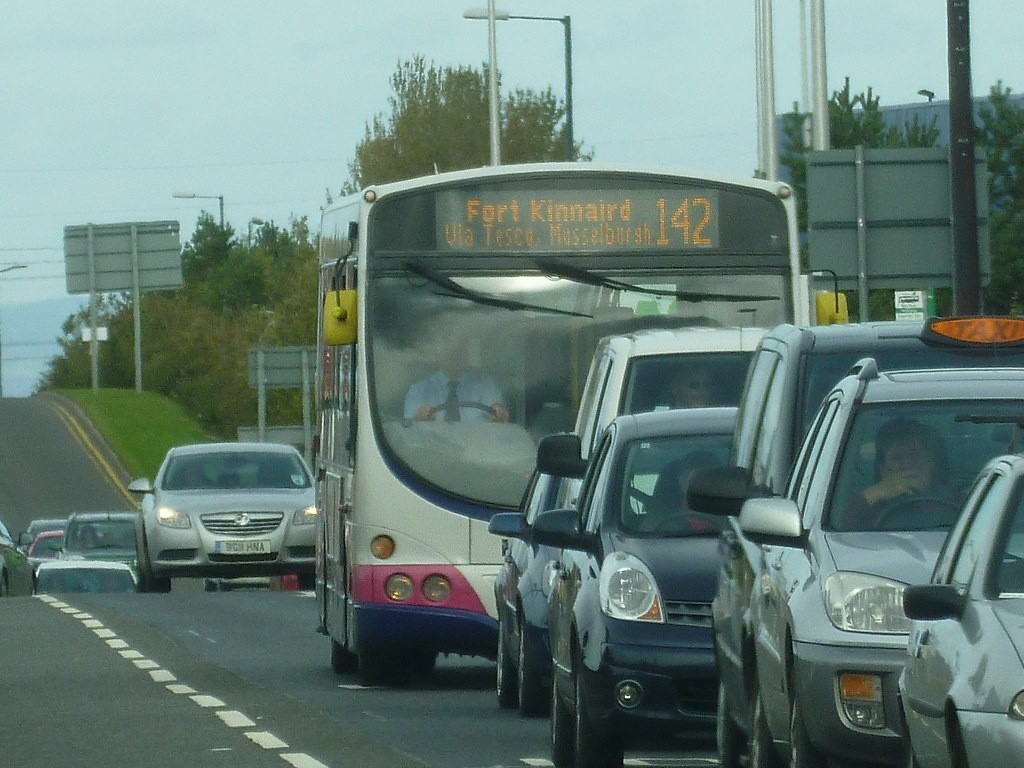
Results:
[127,441,318,593]
[485,462,564,714]
[0,511,140,598]
[895,451,1024,767]
[531,407,741,768]
[202,576,271,593]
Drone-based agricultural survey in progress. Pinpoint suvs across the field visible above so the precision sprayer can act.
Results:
[688,313,1024,767]
[734,356,1024,768]
[531,324,774,513]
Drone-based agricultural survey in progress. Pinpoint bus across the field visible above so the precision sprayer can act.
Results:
[317,161,850,690]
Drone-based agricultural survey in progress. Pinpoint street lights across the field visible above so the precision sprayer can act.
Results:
[465,9,573,163]
[169,191,225,230]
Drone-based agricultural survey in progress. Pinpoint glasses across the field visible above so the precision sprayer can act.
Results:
[681,375,715,392]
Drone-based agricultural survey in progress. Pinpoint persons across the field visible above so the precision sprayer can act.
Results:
[180,460,216,490]
[402,341,510,427]
[675,362,716,410]
[840,416,961,529]
[640,447,723,532]
[81,525,101,548]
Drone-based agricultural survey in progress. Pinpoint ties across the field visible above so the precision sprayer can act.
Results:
[442,379,464,423]
